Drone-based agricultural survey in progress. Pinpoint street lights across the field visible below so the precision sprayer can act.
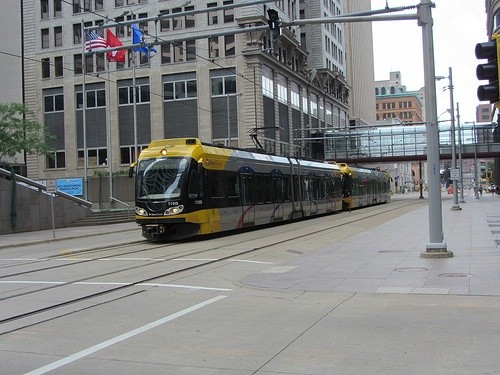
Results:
[435,67,462,210]
[465,121,479,199]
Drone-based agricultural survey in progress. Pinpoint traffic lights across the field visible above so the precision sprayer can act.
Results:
[267,9,278,30]
[475,34,500,112]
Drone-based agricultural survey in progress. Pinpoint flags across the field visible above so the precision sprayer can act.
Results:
[132,23,157,57]
[106,27,128,62]
[85,29,107,51]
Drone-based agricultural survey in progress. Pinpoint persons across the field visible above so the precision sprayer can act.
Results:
[479,185,484,197]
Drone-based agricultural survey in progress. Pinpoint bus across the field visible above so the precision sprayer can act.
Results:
[128,127,393,242]
